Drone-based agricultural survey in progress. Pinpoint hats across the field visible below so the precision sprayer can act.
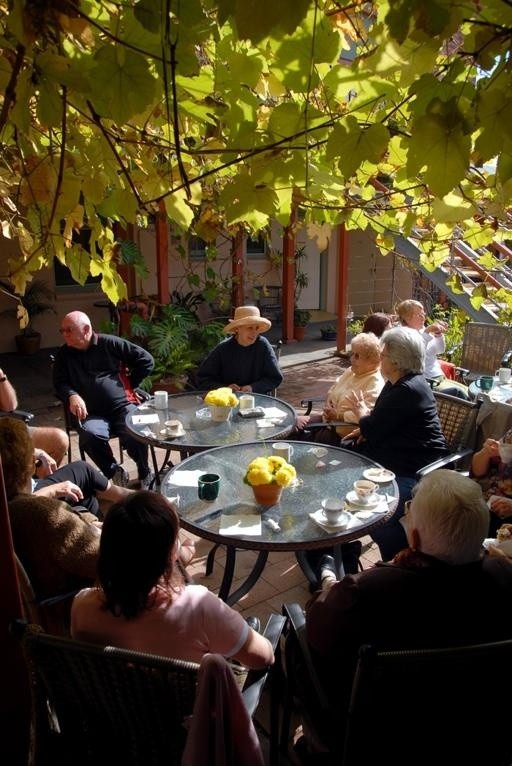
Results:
[222,305,273,335]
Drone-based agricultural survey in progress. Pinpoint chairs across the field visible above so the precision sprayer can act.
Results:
[253,285,282,326]
[0,316,511,766]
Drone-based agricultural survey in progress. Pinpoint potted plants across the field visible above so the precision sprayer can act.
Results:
[293,309,312,341]
[0,279,59,357]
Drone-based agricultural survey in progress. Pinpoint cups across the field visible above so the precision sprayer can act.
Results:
[475,375,494,393]
[154,391,168,411]
[240,395,255,409]
[353,479,379,502]
[197,473,222,504]
[165,420,180,434]
[272,442,294,464]
[494,368,512,384]
[320,497,346,523]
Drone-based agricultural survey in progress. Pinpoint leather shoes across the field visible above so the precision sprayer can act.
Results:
[316,554,337,586]
[245,616,261,633]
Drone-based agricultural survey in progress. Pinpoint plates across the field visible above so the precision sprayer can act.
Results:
[314,508,352,526]
[362,468,396,483]
[346,490,381,508]
[159,428,187,438]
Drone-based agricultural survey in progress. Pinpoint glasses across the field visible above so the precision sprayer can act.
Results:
[58,323,85,333]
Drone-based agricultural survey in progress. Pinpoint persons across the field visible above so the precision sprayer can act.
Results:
[0,368,136,517]
[54,310,156,493]
[196,306,283,396]
[473,427,512,561]
[0,416,195,598]
[64,489,275,687]
[289,299,448,502]
[288,471,512,766]
